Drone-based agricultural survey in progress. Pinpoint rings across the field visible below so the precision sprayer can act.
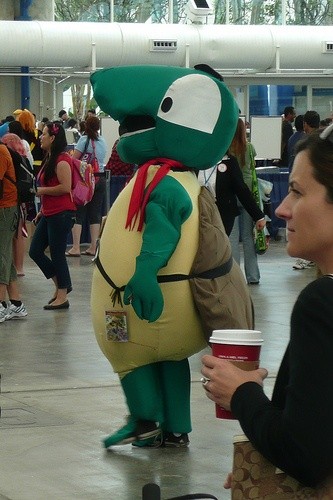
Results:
[201,378,210,386]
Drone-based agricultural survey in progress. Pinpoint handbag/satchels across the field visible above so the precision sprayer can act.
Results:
[73,132,80,143]
[231,440,333,500]
[79,137,100,184]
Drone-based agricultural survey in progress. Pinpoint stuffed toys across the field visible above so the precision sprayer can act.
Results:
[90,64,240,449]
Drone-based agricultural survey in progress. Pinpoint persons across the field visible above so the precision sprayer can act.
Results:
[281,106,333,167]
[195,118,267,283]
[200,133,333,500]
[0,109,107,256]
[0,140,28,322]
[106,125,135,208]
[29,122,77,310]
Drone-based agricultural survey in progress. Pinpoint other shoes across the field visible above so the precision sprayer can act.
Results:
[43,300,69,310]
[113,420,160,444]
[48,287,72,304]
[155,429,190,447]
[293,258,316,269]
[0,301,28,322]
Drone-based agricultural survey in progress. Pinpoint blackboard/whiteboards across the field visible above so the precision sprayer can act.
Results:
[100,116,120,166]
[250,114,283,160]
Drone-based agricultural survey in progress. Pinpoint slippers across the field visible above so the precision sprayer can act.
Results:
[81,251,96,256]
[65,251,81,257]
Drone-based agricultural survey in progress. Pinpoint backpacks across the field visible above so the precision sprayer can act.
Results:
[0,141,38,203]
[58,151,96,207]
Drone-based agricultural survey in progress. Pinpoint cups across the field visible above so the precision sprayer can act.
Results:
[208,329,265,421]
